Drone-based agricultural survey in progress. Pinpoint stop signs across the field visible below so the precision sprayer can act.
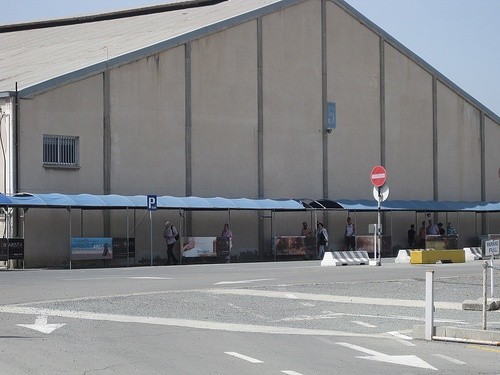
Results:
[370,165,387,187]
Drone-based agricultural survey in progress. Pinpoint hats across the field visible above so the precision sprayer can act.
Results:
[165,221,169,225]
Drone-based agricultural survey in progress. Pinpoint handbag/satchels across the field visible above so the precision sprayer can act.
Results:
[171,225,179,240]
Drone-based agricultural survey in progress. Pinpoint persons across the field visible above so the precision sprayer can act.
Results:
[315,223,329,259]
[221,223,232,239]
[163,220,178,266]
[344,217,356,251]
[301,222,312,236]
[407,220,457,248]
